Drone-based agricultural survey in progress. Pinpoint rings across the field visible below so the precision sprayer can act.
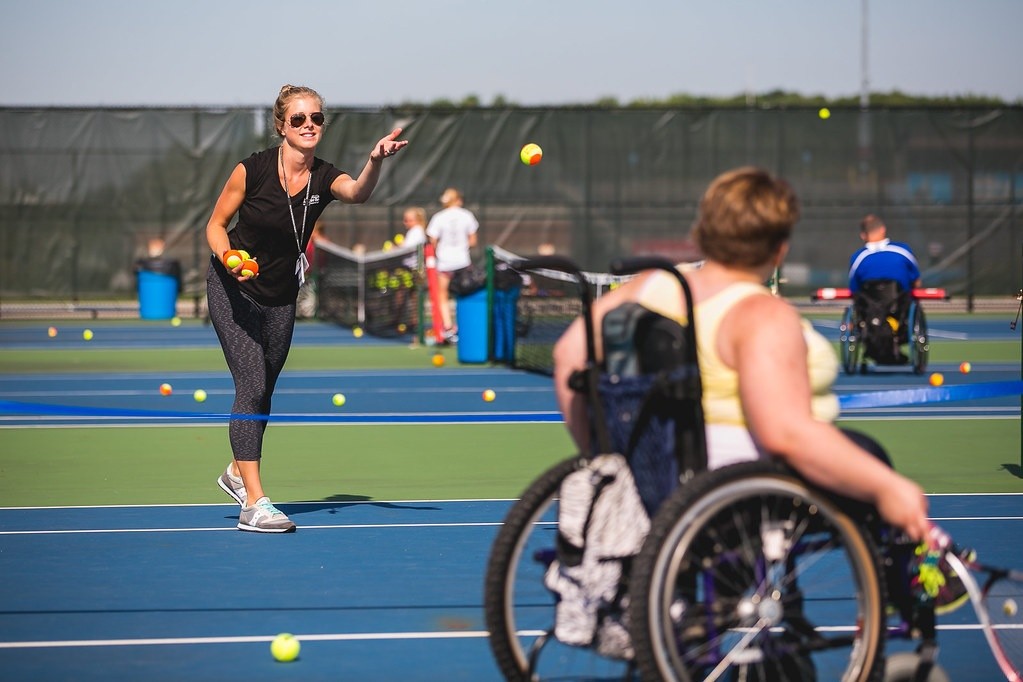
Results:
[386,149,394,155]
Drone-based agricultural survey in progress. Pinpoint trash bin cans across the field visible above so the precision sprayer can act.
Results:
[132,255,183,321]
[447,265,522,365]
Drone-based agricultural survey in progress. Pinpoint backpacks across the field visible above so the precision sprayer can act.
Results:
[544,363,692,662]
[850,289,909,368]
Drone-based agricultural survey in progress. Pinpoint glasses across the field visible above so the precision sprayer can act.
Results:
[282,112,324,128]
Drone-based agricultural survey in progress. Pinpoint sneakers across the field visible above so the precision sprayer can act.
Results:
[873,543,977,624]
[236,496,295,535]
[432,326,459,344]
[217,461,248,506]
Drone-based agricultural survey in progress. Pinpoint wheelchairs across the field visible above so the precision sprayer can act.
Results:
[838,265,929,379]
[483,249,1020,682]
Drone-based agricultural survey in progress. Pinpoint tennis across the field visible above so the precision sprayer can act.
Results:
[240,260,258,277]
[930,372,943,386]
[398,323,406,332]
[432,354,443,366]
[224,250,241,268]
[47,326,58,338]
[171,317,182,326]
[332,394,345,406]
[160,383,172,395]
[521,143,542,165]
[270,631,300,661]
[960,362,971,374]
[353,327,364,337]
[237,249,250,261]
[83,329,93,339]
[193,389,206,402]
[483,389,495,401]
[820,108,830,119]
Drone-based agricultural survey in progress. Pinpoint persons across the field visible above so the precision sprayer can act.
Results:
[845,215,921,300]
[201,82,410,535]
[426,187,480,340]
[550,167,958,681]
[400,202,428,333]
